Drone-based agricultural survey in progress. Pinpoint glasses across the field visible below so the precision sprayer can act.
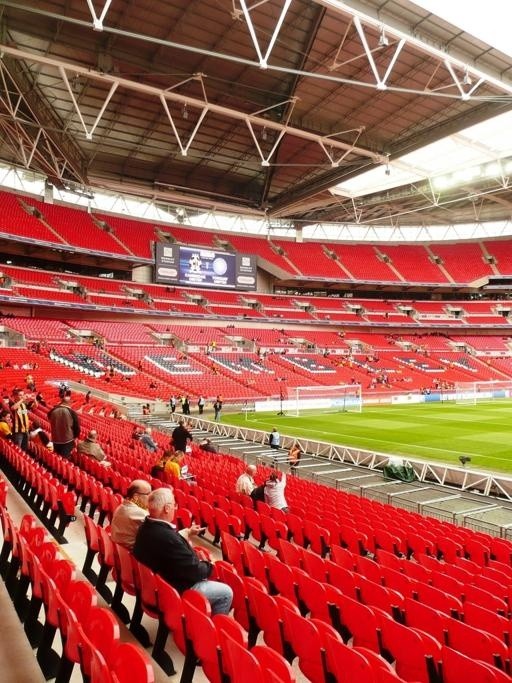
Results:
[166,503,179,510]
[136,492,150,496]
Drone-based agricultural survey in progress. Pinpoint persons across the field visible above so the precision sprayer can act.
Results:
[112,479,207,551]
[1,271,512,512]
[136,487,234,616]
